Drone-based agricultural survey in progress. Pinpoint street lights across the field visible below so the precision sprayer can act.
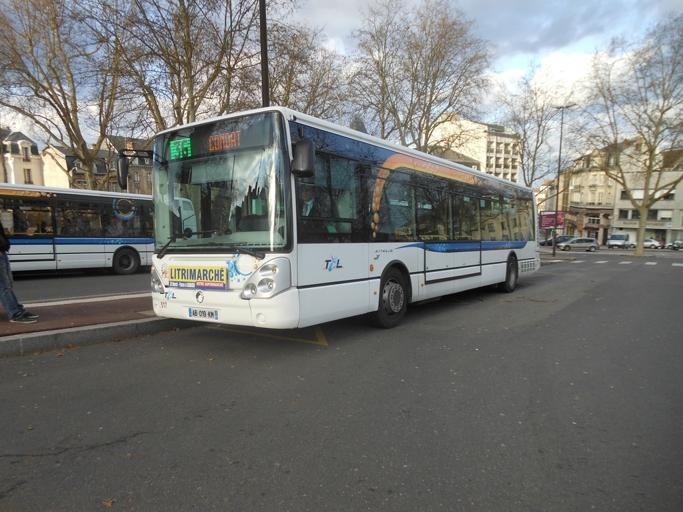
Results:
[548,102,576,257]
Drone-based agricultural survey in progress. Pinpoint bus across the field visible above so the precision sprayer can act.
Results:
[111,104,543,332]
[0,181,199,278]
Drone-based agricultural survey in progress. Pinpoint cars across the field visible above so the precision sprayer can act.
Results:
[538,232,682,253]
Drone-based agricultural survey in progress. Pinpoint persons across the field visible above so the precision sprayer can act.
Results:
[0,221,39,324]
[13,208,123,236]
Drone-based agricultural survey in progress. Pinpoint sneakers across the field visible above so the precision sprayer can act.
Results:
[9,310,39,324]
